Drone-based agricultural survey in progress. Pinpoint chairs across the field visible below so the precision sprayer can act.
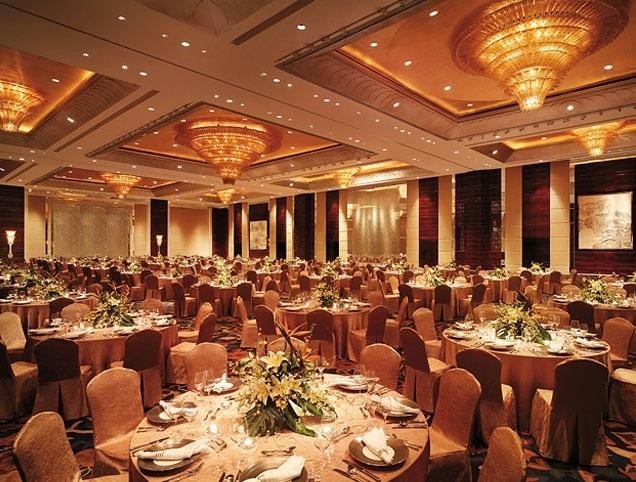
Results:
[0,256,636,435]
[86,367,145,464]
[423,370,482,477]
[527,356,611,464]
[10,409,130,482]
[476,426,530,482]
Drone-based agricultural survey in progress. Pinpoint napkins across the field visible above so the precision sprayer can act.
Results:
[247,454,305,482]
[359,428,395,466]
[134,441,209,463]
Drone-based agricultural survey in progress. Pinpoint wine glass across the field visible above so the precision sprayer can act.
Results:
[465,310,588,337]
[164,354,392,477]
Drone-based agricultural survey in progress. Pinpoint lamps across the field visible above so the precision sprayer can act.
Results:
[332,166,362,188]
[170,118,284,184]
[0,79,44,133]
[451,1,630,117]
[6,230,17,259]
[154,235,164,256]
[98,171,144,204]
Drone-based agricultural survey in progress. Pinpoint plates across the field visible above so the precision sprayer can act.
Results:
[280,299,372,313]
[0,291,89,305]
[446,322,608,355]
[31,311,175,341]
[136,375,419,481]
[551,294,634,308]
